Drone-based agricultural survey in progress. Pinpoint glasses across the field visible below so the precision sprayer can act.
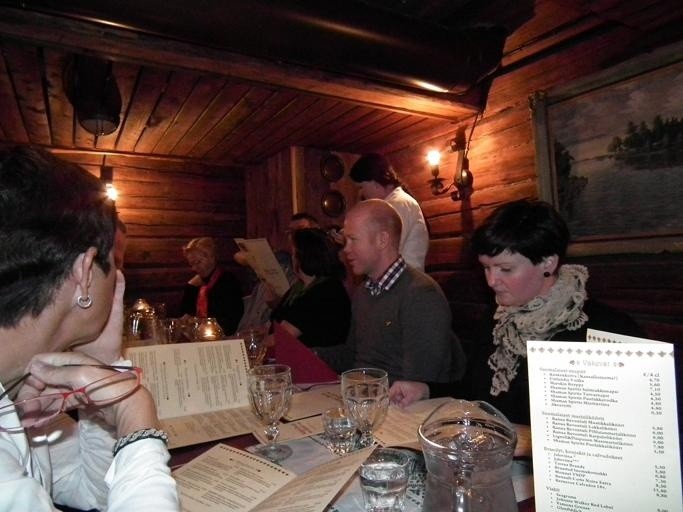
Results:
[0,363,144,434]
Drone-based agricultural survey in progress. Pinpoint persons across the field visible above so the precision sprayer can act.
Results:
[164,151,642,427]
[0,140,180,512]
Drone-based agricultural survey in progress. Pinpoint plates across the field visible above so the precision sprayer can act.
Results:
[320,154,345,181]
[324,223,346,252]
[322,190,346,217]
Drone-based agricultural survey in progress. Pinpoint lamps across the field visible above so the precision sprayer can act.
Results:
[426,137,472,201]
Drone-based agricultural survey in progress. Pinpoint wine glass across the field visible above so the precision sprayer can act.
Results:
[341,367,391,451]
[248,363,294,463]
[122,300,269,370]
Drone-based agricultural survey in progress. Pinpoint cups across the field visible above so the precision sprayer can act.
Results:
[322,408,359,457]
[358,450,410,510]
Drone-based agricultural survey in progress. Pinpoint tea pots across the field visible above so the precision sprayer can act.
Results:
[419,398,519,512]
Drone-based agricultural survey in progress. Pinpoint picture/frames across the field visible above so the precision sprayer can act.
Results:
[528,39,683,259]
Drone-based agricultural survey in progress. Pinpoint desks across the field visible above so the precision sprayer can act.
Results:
[162,334,535,512]
